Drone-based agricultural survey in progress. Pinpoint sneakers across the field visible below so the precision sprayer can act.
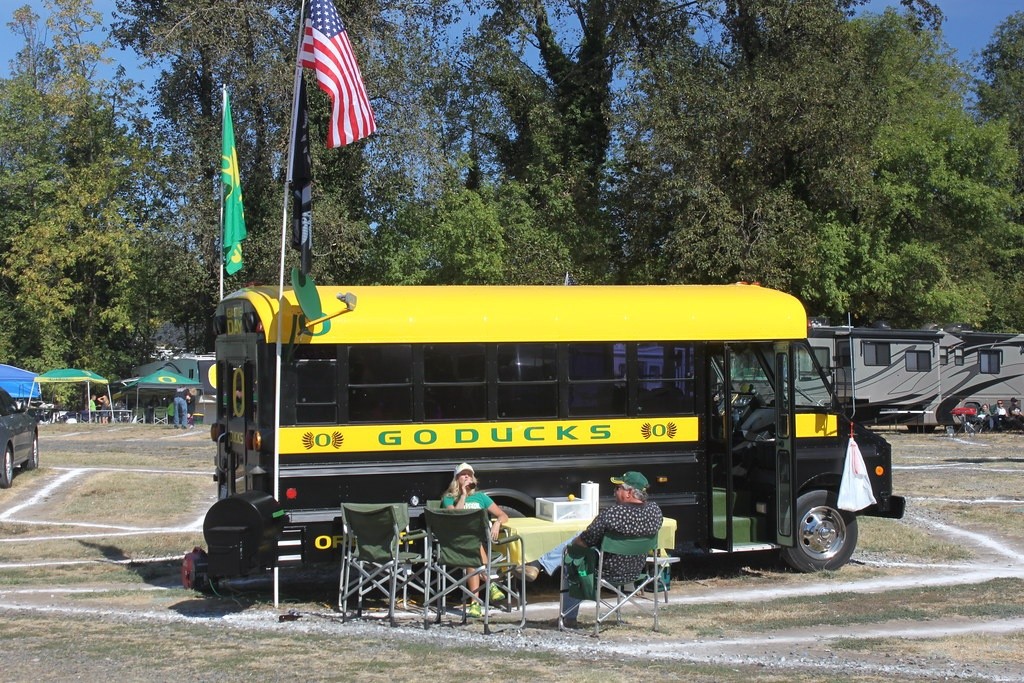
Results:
[466,601,483,619]
[487,585,506,602]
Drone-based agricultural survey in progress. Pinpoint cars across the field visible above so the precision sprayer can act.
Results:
[0,386,39,490]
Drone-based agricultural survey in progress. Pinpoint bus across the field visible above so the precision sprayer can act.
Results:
[178,282,907,601]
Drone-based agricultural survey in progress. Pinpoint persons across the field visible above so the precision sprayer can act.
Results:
[98,396,109,423]
[174,386,190,428]
[90,395,96,421]
[978,398,1024,432]
[514,472,663,629]
[148,395,160,421]
[442,464,508,617]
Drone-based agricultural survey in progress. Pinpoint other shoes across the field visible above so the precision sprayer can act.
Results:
[548,617,577,627]
[513,564,539,582]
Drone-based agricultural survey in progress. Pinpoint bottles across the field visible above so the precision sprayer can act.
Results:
[467,483,475,488]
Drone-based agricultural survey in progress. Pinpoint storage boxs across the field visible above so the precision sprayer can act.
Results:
[536,497,590,524]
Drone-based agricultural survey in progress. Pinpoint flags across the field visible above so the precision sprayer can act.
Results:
[301,0,377,148]
[288,71,313,275]
[221,96,246,275]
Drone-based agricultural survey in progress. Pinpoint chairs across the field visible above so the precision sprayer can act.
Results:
[560,529,658,637]
[647,386,684,414]
[338,500,527,635]
[132,407,146,424]
[154,406,168,425]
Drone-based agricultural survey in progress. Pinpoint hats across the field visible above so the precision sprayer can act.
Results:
[610,471,649,492]
[453,462,475,477]
[1010,398,1018,403]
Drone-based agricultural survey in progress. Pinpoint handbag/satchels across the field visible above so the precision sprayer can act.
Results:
[836,422,877,513]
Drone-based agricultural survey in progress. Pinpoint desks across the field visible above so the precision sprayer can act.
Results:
[35,408,72,423]
[90,410,132,423]
[491,518,677,610]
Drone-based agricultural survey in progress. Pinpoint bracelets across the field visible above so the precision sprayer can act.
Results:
[497,520,502,526]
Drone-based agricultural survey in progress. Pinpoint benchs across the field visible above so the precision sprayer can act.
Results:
[646,557,680,604]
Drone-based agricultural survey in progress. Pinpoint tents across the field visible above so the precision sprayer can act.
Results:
[121,369,205,423]
[0,364,45,420]
[27,369,114,423]
